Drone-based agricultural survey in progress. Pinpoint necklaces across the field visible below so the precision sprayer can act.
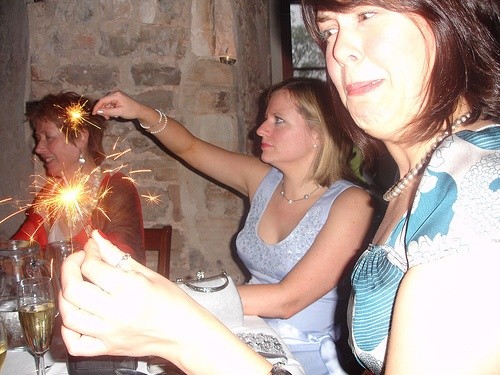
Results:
[47,166,101,214]
[279,176,323,205]
[382,108,476,202]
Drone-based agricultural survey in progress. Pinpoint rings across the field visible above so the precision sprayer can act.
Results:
[115,252,131,269]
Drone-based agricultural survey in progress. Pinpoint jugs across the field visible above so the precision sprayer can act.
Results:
[0,240,58,347]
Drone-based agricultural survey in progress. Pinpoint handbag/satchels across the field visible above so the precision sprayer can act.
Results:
[170,269,244,330]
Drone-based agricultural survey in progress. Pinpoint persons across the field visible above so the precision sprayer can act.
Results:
[57,0,498,375]
[91,77,384,375]
[0,88,146,279]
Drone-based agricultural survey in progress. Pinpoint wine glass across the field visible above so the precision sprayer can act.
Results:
[17,277,55,375]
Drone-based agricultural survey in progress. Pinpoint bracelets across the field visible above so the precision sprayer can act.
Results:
[139,109,163,130]
[144,112,167,134]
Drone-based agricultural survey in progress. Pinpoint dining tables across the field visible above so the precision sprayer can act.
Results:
[234,314,303,375]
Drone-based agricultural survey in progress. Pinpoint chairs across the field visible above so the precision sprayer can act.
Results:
[143,226,172,281]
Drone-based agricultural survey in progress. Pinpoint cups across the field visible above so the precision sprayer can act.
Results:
[0,315,9,375]
[46,241,79,281]
